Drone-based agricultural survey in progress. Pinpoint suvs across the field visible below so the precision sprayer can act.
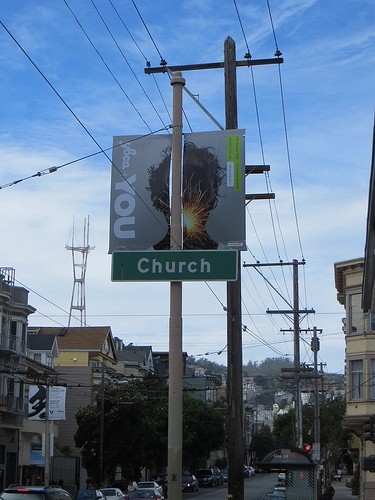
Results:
[0,483,73,500]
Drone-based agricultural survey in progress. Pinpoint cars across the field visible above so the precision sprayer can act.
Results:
[182,473,199,492]
[245,465,254,477]
[76,489,105,500]
[100,488,126,500]
[133,482,162,493]
[122,488,164,500]
[278,473,285,481]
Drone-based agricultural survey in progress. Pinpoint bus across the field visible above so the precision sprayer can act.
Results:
[195,469,224,486]
